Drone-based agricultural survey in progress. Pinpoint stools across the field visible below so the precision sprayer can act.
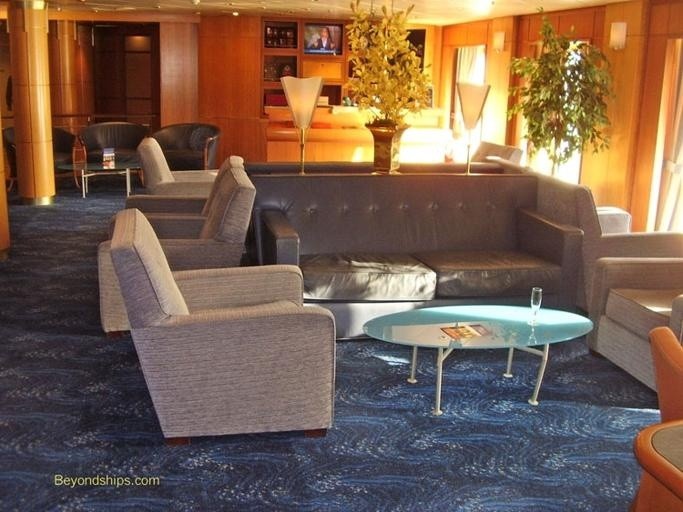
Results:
[632,418,683,512]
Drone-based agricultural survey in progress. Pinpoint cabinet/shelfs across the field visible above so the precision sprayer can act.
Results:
[197,13,443,172]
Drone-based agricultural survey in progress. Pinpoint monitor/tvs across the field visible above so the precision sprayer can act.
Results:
[303,23,343,55]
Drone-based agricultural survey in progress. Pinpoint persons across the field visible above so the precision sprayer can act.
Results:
[309,27,335,50]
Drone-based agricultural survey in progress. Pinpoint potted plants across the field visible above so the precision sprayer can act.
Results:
[342,0,430,177]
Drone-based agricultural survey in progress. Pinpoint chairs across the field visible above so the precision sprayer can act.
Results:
[151,123,221,170]
[470,142,524,162]
[77,121,149,161]
[2,127,75,192]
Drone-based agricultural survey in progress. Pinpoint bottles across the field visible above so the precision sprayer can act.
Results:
[264,25,296,46]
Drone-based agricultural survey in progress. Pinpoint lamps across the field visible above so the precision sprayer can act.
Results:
[280,76,324,177]
[492,31,505,53]
[457,81,491,176]
[609,21,627,50]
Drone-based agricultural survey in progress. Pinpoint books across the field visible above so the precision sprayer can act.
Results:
[102,147,114,169]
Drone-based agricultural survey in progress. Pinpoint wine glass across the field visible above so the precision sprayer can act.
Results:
[530,287,543,327]
